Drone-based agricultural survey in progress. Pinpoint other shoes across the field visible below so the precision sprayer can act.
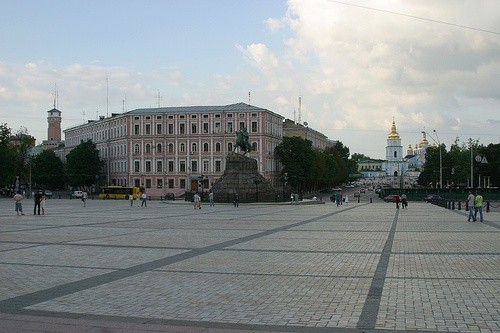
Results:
[473,219,477,222]
[468,219,470,222]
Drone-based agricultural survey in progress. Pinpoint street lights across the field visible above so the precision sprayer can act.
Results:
[421,129,442,189]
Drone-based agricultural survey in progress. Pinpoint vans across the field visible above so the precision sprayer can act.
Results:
[74,190,83,199]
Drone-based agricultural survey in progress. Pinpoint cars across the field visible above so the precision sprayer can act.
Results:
[39,190,52,199]
[344,180,382,194]
[424,194,441,203]
[354,191,360,197]
[319,186,342,194]
[384,195,402,203]
[360,188,365,193]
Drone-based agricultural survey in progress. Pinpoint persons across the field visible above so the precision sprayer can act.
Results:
[33,191,45,215]
[465,190,476,222]
[81,190,87,207]
[136,192,140,207]
[240,127,249,151]
[234,192,240,208]
[142,192,147,207]
[193,192,202,209]
[330,191,349,207]
[401,193,407,209]
[13,192,24,216]
[474,191,483,222]
[0,188,34,199]
[426,180,455,189]
[290,191,317,201]
[209,190,214,207]
[395,194,400,209]
[129,192,134,208]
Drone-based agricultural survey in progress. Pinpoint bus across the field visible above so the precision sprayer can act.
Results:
[98,185,143,200]
